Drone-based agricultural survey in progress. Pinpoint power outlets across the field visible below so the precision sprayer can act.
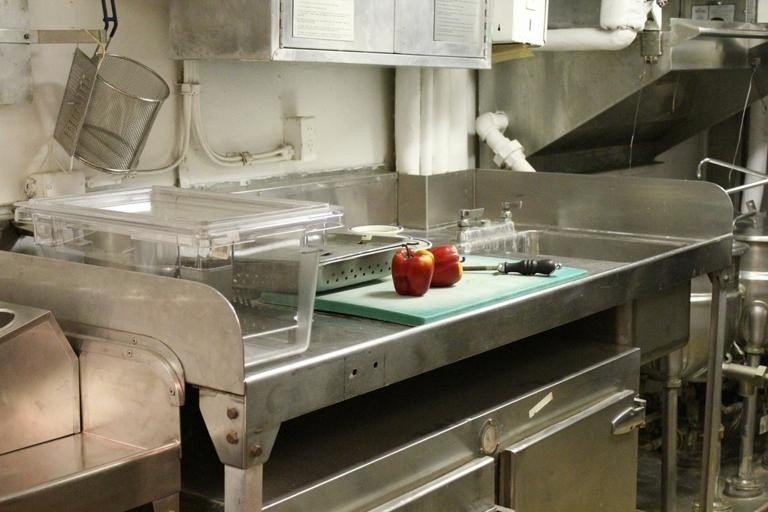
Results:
[282,115,316,160]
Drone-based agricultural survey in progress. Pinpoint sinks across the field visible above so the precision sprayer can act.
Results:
[457,229,691,368]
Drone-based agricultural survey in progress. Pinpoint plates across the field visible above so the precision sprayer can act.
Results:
[348,223,404,237]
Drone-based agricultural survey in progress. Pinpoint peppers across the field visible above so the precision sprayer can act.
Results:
[426,244,466,286]
[392,243,435,296]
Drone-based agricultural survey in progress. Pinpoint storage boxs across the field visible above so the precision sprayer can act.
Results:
[15,185,344,366]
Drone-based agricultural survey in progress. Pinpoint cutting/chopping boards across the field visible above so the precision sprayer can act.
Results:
[259,253,588,325]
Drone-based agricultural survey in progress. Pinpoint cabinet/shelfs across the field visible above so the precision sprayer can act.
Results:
[260,348,646,511]
[168,0,495,71]
